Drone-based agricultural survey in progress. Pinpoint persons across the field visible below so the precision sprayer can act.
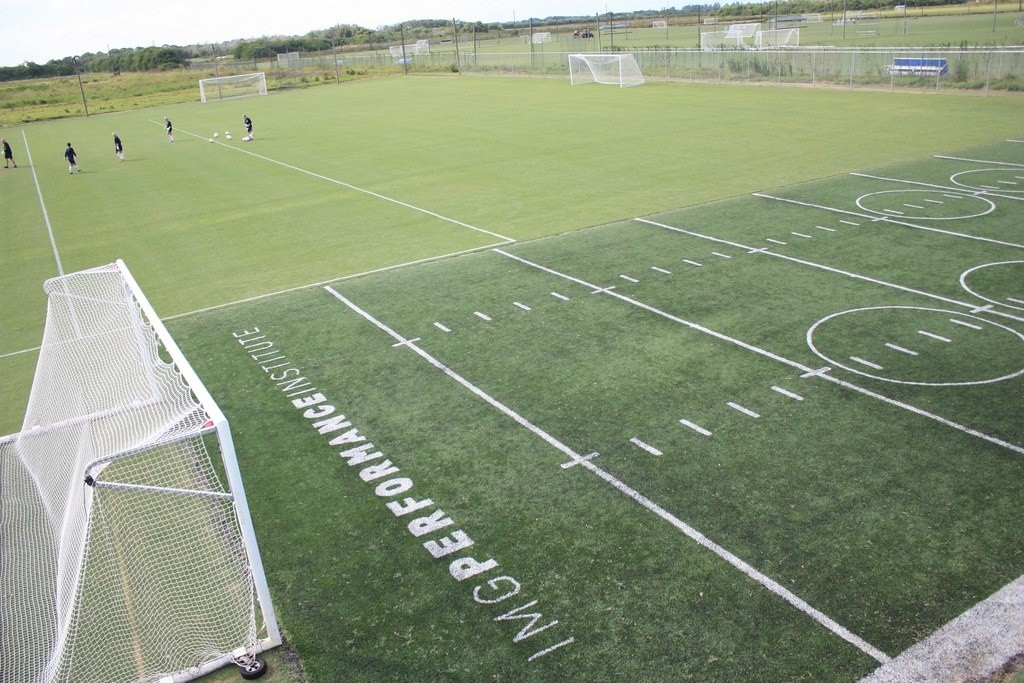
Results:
[112,132,125,161]
[1,138,18,168]
[164,117,174,142]
[243,114,254,140]
[64,143,81,174]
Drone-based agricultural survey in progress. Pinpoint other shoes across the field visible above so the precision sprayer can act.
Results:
[13,166,17,168]
[78,169,80,172]
[70,172,73,174]
[4,166,8,168]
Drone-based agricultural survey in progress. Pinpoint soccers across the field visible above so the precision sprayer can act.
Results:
[209,139,213,143]
[225,131,232,140]
[243,137,250,141]
[214,132,219,138]
